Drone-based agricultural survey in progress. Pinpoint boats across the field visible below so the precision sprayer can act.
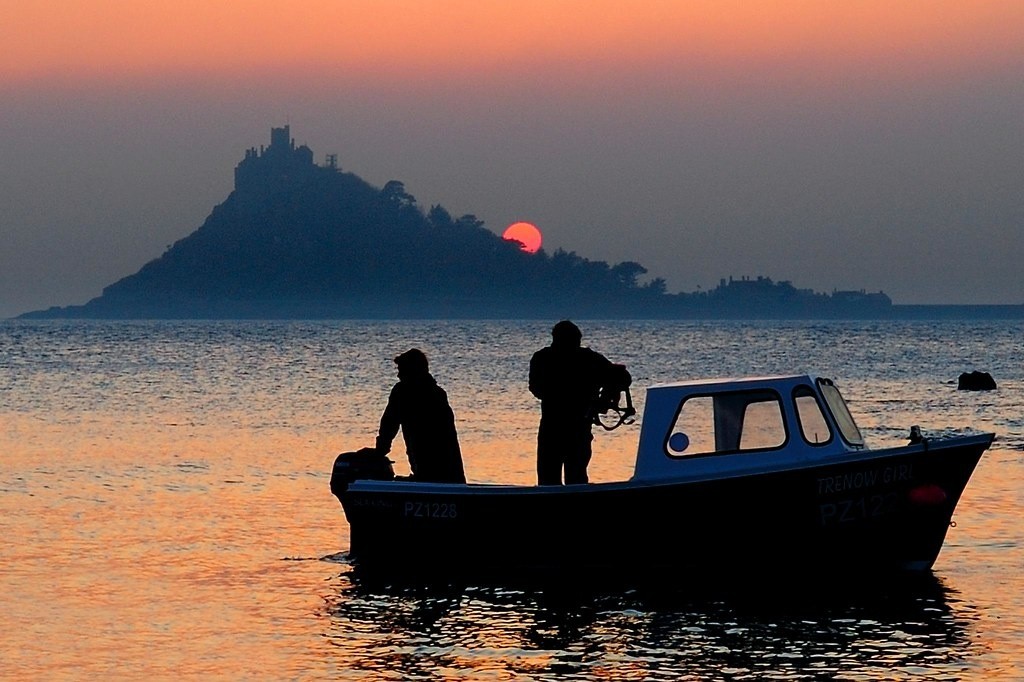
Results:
[329,373,997,593]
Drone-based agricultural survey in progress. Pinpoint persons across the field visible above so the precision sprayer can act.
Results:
[526,319,633,489]
[376,346,466,486]
